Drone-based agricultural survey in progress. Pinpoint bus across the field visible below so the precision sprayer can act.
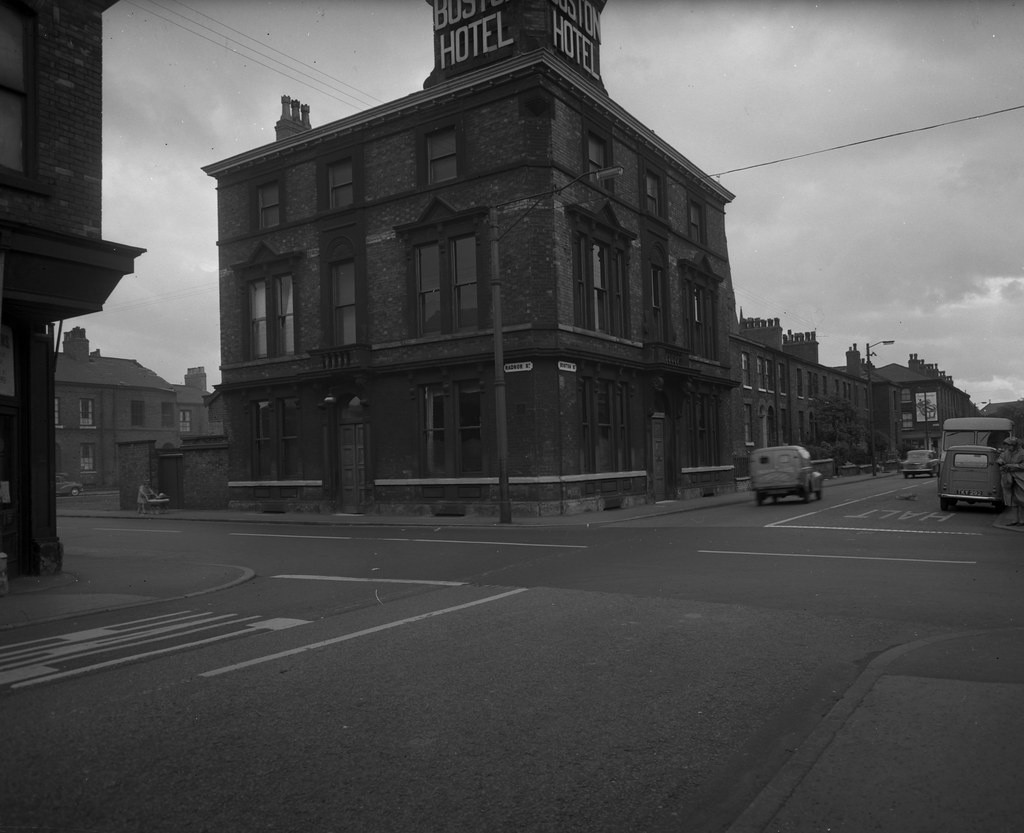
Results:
[941,417,1017,475]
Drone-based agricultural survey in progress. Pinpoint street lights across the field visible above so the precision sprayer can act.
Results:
[865,339,895,479]
[486,164,625,526]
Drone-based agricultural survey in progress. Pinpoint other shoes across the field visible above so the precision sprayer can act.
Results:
[1006,521,1024,527]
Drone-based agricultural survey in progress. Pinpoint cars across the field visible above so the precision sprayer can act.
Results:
[900,449,940,478]
[938,444,1006,515]
[55,476,84,496]
[749,446,825,506]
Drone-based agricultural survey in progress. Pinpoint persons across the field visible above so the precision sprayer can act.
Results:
[996,437,1024,526]
[137,479,154,514]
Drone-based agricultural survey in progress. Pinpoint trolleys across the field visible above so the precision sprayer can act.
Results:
[144,494,171,515]
